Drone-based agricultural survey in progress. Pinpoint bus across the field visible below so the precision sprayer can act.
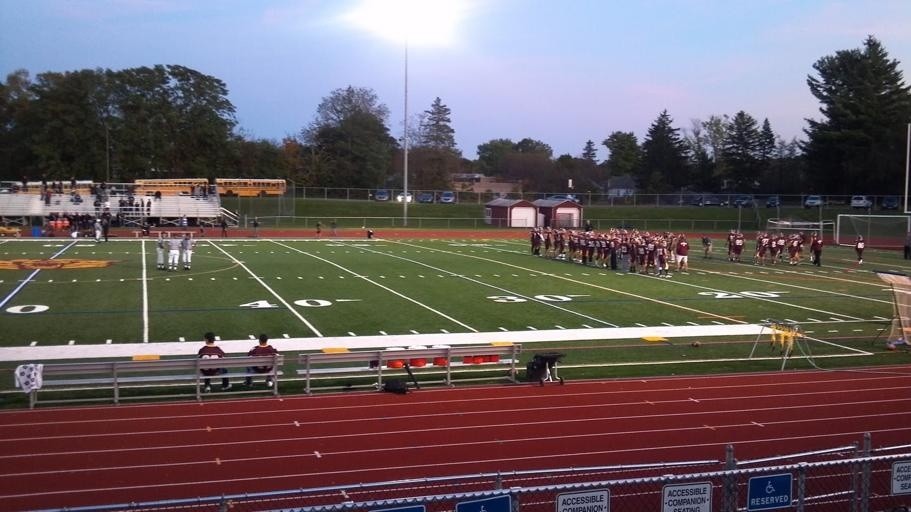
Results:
[134,178,209,195]
[215,178,287,196]
[12,180,95,195]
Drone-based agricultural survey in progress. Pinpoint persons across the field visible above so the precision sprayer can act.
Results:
[242,333,279,388]
[22,175,30,192]
[166,233,184,271]
[331,218,337,237]
[855,234,867,265]
[221,219,229,237]
[317,220,323,237]
[585,218,593,231]
[197,331,234,393]
[156,231,167,269]
[727,228,825,269]
[34,176,209,244]
[253,216,259,236]
[181,232,197,269]
[701,235,713,260]
[530,224,692,279]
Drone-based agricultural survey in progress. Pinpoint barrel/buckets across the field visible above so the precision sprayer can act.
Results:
[408,346,427,366]
[386,347,406,367]
[431,345,450,368]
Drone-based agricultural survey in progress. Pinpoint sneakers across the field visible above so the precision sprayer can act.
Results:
[202,379,277,393]
[155,263,193,272]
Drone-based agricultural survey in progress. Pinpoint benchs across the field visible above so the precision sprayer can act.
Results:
[2,180,222,232]
[0,343,525,414]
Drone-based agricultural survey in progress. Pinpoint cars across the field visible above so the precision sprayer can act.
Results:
[417,192,434,203]
[440,191,455,202]
[375,188,390,200]
[766,197,783,208]
[881,197,899,209]
[850,196,872,210]
[691,195,728,207]
[546,194,583,204]
[733,195,752,208]
[396,193,414,203]
[805,195,823,207]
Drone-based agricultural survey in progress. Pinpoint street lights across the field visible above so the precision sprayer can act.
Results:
[391,6,418,228]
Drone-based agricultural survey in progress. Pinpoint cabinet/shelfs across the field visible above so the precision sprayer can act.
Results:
[751,319,826,374]
